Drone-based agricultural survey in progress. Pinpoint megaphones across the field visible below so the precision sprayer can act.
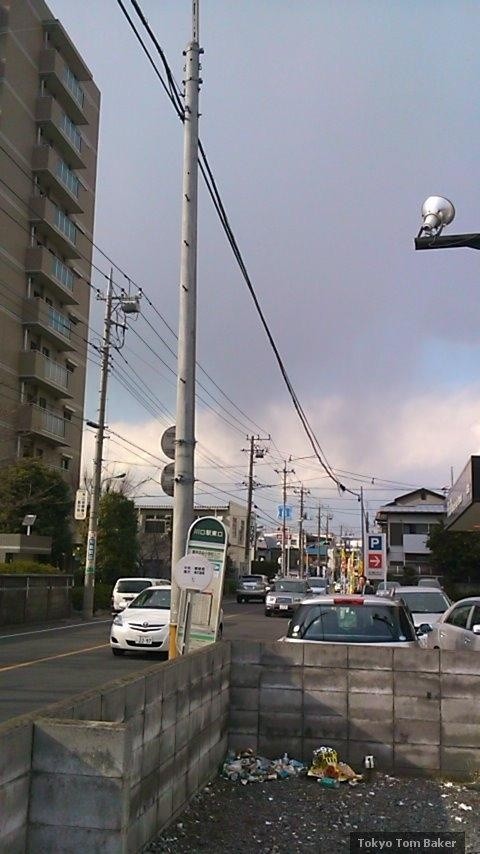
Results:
[422,195,455,231]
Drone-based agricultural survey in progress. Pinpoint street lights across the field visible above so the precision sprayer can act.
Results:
[78,471,127,618]
[407,189,480,254]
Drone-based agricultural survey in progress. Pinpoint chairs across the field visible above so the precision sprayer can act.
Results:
[312,610,392,635]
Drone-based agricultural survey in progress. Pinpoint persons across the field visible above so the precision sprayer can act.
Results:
[353,575,373,594]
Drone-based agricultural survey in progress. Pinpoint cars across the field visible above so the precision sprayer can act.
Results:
[106,584,226,661]
[424,595,480,651]
[234,571,459,651]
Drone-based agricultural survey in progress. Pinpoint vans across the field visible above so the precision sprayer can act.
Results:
[108,576,172,617]
